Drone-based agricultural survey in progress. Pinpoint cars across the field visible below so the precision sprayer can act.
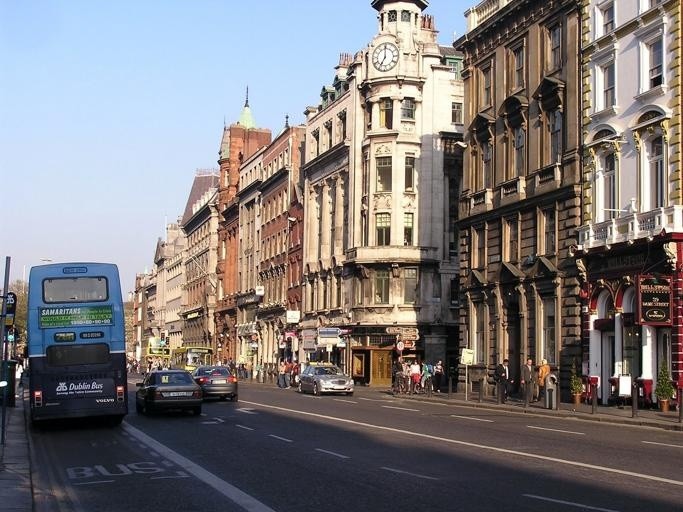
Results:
[297,365,355,397]
[136,370,203,416]
[194,366,238,402]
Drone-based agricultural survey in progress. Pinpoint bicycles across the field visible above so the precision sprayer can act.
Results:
[413,375,433,397]
[393,373,405,395]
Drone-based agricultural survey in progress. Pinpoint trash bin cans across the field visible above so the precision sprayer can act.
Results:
[5,360,18,408]
[544,373,558,409]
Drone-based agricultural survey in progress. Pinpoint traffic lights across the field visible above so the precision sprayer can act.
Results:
[166,336,169,345]
[161,333,165,343]
[287,337,292,349]
[8,329,15,341]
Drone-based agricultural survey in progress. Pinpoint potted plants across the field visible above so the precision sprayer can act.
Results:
[570,375,583,404]
[654,360,674,412]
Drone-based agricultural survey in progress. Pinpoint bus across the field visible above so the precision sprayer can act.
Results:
[28,264,129,428]
[147,337,171,368]
[171,348,213,370]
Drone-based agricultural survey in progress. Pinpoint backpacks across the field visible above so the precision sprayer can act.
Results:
[427,365,433,374]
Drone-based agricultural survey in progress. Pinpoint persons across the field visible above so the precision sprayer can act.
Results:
[538,358,550,401]
[494,360,514,402]
[392,359,446,393]
[126,355,250,381]
[278,357,310,389]
[521,359,536,406]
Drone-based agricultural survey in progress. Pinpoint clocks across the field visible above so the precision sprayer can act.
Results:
[372,42,399,71]
